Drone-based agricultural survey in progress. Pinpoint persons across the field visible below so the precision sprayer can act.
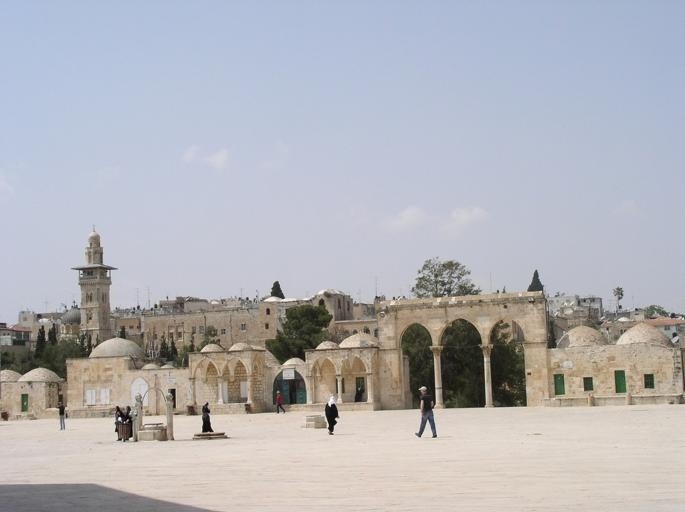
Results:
[276,391,285,413]
[415,386,437,438]
[355,386,364,402]
[56,402,65,430]
[325,396,338,435]
[202,402,214,433]
[115,406,132,441]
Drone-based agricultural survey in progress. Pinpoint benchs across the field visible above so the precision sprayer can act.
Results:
[192,433,227,440]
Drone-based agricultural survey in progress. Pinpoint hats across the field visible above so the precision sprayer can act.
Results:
[418,385,428,392]
[276,390,280,394]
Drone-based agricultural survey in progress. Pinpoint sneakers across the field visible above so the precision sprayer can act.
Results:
[432,434,438,438]
[414,432,421,438]
[328,430,334,436]
